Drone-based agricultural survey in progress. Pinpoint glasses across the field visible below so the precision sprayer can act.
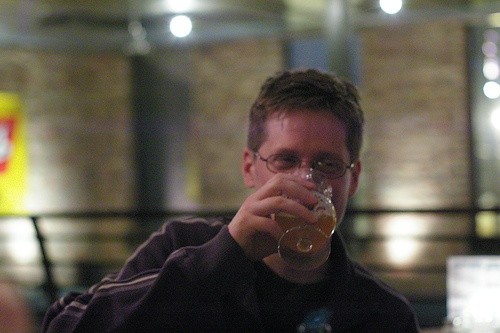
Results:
[253,150,354,178]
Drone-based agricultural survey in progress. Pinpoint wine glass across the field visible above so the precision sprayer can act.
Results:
[271,168,336,269]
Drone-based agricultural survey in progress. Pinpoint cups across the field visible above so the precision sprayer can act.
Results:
[445,255,500,333]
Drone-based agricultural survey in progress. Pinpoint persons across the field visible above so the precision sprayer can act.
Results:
[41,68,421,333]
